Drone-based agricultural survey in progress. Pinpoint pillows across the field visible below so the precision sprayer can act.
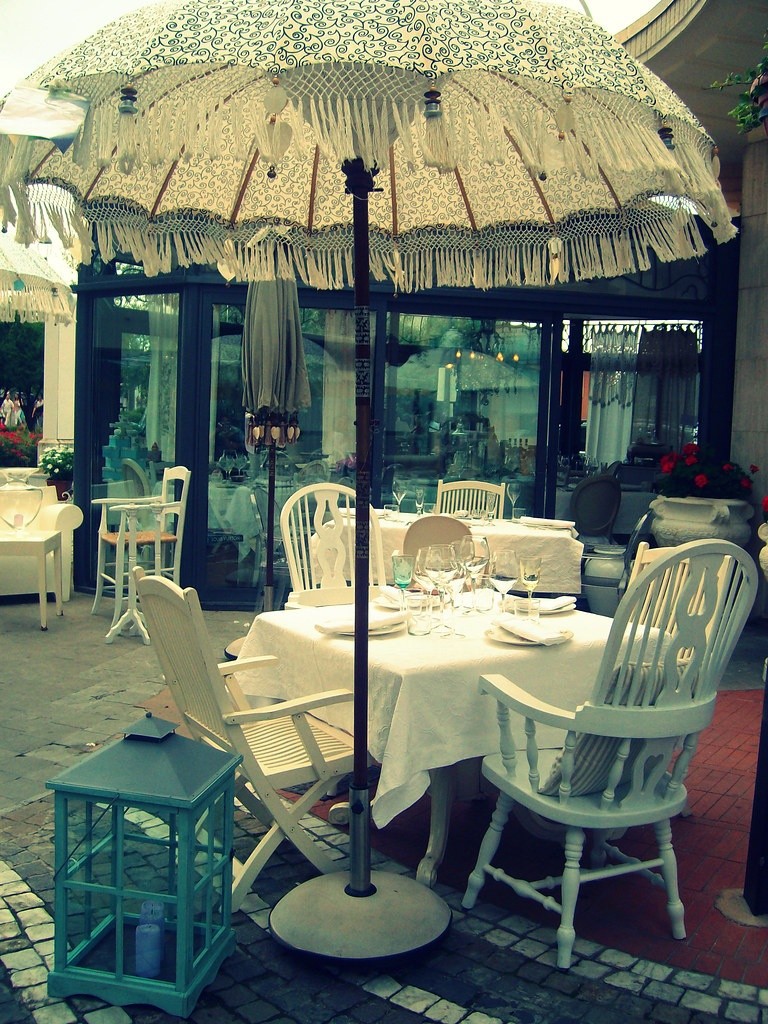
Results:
[539,653,712,798]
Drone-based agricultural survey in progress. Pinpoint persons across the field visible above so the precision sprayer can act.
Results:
[215,414,242,459]
[0,392,44,432]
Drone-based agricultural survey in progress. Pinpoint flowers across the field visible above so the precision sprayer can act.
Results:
[761,494,768,524]
[37,444,74,481]
[660,443,759,500]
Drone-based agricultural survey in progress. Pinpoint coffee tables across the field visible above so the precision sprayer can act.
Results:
[0,527,63,632]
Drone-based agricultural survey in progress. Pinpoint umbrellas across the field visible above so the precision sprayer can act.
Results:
[238,241,313,446]
[0,0,740,813]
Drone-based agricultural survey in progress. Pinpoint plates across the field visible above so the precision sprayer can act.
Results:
[316,604,405,636]
[484,619,575,646]
[506,593,578,614]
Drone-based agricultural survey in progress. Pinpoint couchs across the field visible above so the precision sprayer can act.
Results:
[0,486,84,602]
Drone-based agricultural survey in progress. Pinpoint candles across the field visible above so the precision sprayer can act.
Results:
[138,900,166,951]
[437,367,445,402]
[135,923,161,977]
[450,371,457,402]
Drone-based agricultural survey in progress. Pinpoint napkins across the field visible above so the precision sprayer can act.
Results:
[381,586,411,608]
[314,610,413,635]
[491,612,567,646]
[519,516,576,527]
[538,596,576,611]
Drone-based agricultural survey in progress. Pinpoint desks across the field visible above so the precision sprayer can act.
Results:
[151,479,316,572]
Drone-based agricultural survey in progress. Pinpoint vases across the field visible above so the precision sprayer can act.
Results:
[46,476,72,501]
[649,494,754,548]
[583,547,630,617]
[757,521,768,584]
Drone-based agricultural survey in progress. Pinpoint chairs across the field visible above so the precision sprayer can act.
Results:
[434,480,506,520]
[249,487,312,587]
[148,450,332,483]
[471,542,737,845]
[603,460,622,478]
[463,537,759,970]
[279,483,390,798]
[131,565,378,913]
[121,458,173,575]
[571,474,622,559]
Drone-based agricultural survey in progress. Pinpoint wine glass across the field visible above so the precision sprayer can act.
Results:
[392,480,408,513]
[507,483,521,522]
[209,446,299,489]
[432,415,601,489]
[391,534,542,638]
[415,486,425,517]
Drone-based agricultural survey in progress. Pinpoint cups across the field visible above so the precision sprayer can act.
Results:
[383,504,399,521]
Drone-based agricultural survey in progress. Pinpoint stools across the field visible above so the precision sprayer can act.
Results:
[91,465,192,629]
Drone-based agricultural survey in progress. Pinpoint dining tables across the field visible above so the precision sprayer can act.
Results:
[311,508,585,598]
[225,588,673,889]
